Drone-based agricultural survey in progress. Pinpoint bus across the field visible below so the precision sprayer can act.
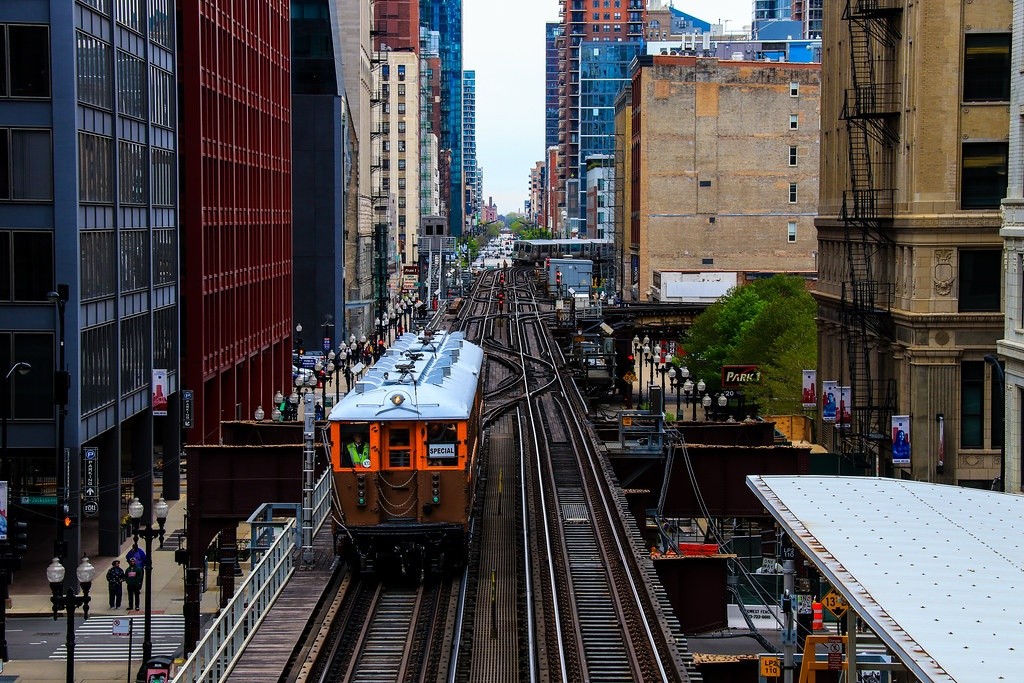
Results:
[512,238,614,264]
[327,326,488,581]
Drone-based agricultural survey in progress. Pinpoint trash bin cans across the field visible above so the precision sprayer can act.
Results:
[120,514,141,545]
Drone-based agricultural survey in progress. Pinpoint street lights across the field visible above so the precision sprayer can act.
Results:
[255,407,281,521]
[315,362,334,420]
[127,497,171,683]
[274,391,299,420]
[328,350,347,404]
[669,365,689,408]
[703,394,727,421]
[644,345,661,384]
[340,341,357,393]
[45,557,95,683]
[633,335,650,410]
[684,379,706,422]
[47,292,67,611]
[375,292,419,347]
[350,334,367,378]
[0,362,33,663]
[298,376,317,393]
[654,353,672,412]
[296,323,302,373]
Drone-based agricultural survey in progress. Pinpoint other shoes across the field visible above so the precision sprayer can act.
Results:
[135,607,139,611]
[111,607,115,610]
[117,607,121,610]
[126,607,133,610]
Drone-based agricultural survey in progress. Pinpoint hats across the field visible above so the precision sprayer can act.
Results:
[130,558,136,562]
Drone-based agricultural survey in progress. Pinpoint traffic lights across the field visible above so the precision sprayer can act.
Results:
[500,274,503,286]
[628,354,634,372]
[499,294,503,312]
[556,271,560,289]
[546,258,550,271]
[0,542,13,588]
[12,520,28,562]
[300,348,304,354]
[65,516,77,526]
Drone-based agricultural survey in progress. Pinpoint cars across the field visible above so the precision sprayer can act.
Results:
[292,364,313,382]
[478,235,513,258]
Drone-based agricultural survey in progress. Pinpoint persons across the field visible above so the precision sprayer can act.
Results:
[396,323,404,335]
[823,393,836,417]
[892,430,910,459]
[315,402,322,420]
[346,343,370,364]
[106,560,125,610]
[125,544,146,595]
[125,558,142,611]
[343,430,370,468]
